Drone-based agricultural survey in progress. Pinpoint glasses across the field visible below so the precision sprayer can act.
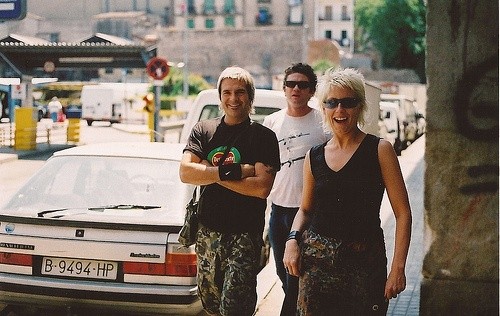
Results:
[285,80,311,91]
[325,96,360,109]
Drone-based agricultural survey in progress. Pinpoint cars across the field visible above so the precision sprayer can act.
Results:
[1,140,272,316]
[35,105,46,123]
[66,105,83,119]
[157,97,189,123]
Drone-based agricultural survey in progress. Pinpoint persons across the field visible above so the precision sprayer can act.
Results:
[179,64,281,316]
[283,65,412,316]
[47,96,62,122]
[262,62,332,316]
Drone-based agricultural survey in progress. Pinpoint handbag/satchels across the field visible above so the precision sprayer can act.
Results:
[177,199,200,247]
[301,230,342,268]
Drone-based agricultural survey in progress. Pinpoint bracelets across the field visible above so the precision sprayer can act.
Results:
[286,232,302,245]
[219,164,242,181]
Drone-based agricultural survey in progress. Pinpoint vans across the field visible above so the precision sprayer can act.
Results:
[179,87,320,146]
[379,93,428,152]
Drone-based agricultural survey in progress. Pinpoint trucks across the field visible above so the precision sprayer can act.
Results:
[80,82,152,127]
[271,72,396,151]
